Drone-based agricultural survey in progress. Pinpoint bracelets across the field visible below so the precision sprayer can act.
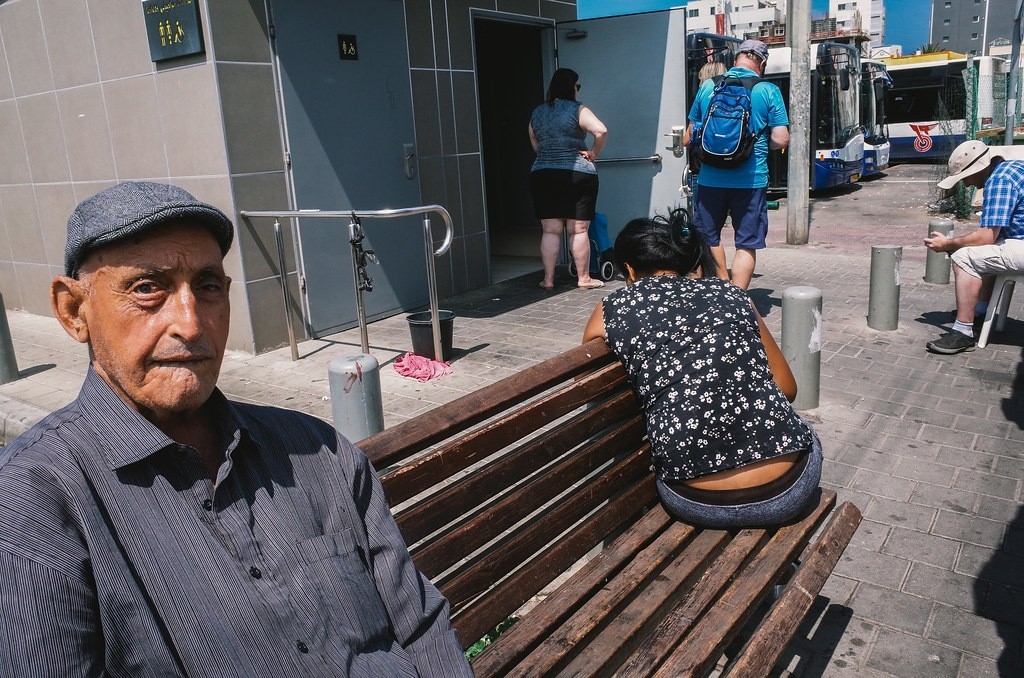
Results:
[591,149,597,156]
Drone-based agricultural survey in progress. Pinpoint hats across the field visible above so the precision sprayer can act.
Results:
[734,39,769,66]
[61,180,235,278]
[937,139,992,190]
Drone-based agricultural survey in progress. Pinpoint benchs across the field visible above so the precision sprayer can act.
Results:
[351,337,864,678]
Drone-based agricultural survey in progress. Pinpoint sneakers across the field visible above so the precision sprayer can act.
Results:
[926,328,976,354]
[950,308,987,329]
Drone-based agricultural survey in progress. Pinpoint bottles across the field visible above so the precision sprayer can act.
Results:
[692,122,704,132]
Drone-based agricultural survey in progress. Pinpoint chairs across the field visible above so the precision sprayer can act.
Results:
[979,270,1024,349]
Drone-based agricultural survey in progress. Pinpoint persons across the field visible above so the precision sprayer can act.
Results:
[683,40,789,292]
[582,202,823,527]
[529,68,608,290]
[924,140,1024,354]
[0,182,474,678]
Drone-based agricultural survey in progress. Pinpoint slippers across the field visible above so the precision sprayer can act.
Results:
[539,281,555,291]
[578,279,605,291]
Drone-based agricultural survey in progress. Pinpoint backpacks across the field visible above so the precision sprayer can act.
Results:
[690,75,767,169]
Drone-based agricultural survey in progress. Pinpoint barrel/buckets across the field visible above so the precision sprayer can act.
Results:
[405,310,456,361]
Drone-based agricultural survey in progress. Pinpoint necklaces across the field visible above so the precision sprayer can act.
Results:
[661,272,678,275]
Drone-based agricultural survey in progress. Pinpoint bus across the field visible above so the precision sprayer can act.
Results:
[688,32,747,125]
[858,57,894,176]
[874,51,1009,165]
[761,41,865,195]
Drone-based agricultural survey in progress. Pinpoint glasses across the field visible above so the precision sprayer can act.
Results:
[575,84,582,92]
